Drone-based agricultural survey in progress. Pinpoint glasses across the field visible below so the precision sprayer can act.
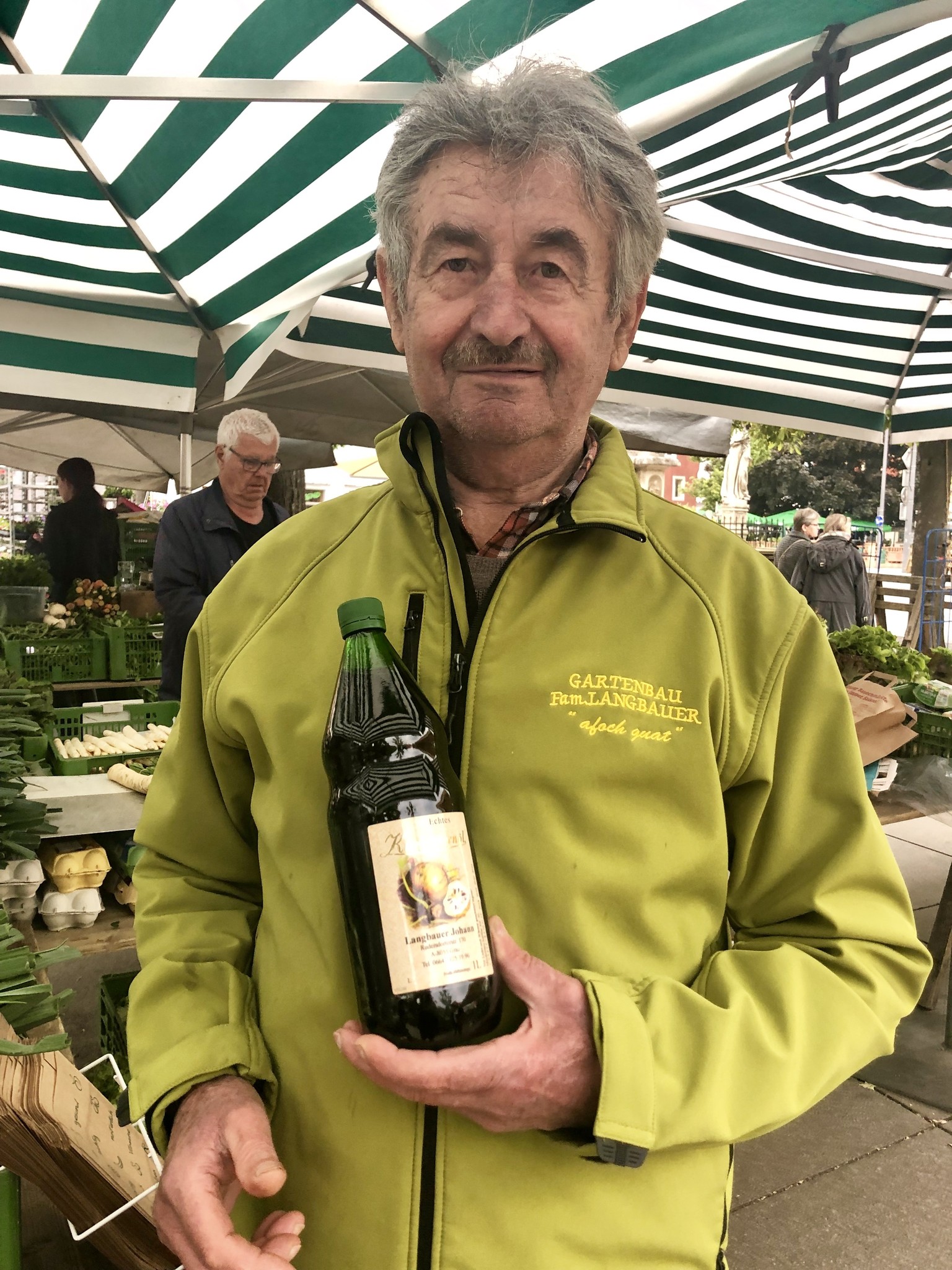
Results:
[224,444,282,474]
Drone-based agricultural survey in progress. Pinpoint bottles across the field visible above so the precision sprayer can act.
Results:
[320,597,504,1050]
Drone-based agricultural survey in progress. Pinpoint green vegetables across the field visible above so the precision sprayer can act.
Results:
[0,611,166,681]
[0,552,59,595]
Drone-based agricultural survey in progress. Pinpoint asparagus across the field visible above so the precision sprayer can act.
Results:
[54,717,177,774]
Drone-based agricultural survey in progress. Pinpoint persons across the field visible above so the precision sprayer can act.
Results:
[42,457,120,611]
[153,409,292,700]
[790,513,875,634]
[773,508,820,594]
[116,60,932,1270]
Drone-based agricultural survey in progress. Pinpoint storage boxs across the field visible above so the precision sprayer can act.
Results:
[44,698,182,778]
[98,969,142,1076]
[890,707,952,760]
[889,680,919,705]
[0,622,109,684]
[85,615,163,681]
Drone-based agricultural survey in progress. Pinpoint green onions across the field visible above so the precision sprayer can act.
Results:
[0,660,83,1055]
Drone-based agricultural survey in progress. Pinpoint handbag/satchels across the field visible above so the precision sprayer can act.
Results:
[846,670,920,767]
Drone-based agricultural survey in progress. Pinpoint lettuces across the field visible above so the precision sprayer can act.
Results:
[811,608,952,688]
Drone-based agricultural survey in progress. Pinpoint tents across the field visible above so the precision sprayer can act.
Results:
[0,0,952,596]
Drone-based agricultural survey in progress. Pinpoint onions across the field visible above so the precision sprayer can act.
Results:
[43,603,76,629]
